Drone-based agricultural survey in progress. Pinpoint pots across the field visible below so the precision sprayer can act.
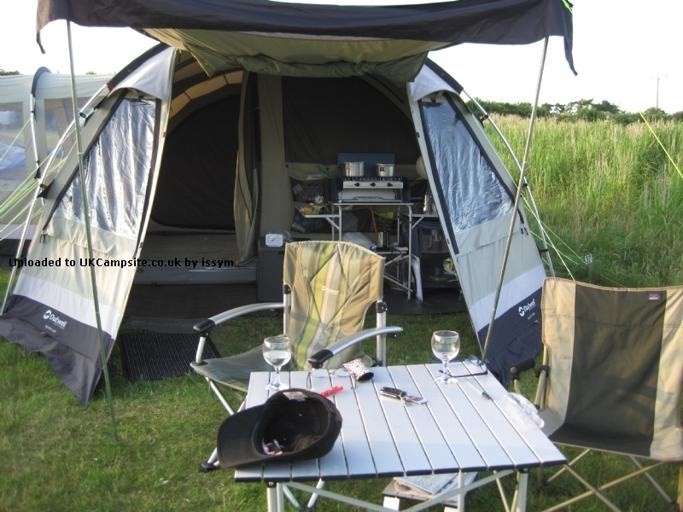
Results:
[344,160,365,178]
[375,161,395,177]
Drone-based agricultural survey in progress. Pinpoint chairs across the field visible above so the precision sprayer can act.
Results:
[190,239,403,471]
[511,277,683,512]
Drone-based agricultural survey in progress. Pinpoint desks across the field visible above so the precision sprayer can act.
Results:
[234,361,567,512]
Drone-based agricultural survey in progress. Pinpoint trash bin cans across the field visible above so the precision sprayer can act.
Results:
[256,235,287,301]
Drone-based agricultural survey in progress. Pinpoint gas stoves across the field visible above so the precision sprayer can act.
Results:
[337,176,404,203]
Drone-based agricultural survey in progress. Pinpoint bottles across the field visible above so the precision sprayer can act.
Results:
[423,183,437,213]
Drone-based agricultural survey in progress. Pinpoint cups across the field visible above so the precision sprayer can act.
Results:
[432,240,442,251]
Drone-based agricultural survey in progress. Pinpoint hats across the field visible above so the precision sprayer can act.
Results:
[217,388,343,469]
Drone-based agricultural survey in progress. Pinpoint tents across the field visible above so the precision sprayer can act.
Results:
[0,0,578,403]
[0,68,120,244]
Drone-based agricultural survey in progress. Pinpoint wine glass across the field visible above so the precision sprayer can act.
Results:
[263,335,292,391]
[431,329,460,385]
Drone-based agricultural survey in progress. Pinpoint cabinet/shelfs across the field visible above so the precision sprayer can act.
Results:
[299,199,438,299]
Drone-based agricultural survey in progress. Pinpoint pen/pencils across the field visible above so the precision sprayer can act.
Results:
[465,380,493,403]
